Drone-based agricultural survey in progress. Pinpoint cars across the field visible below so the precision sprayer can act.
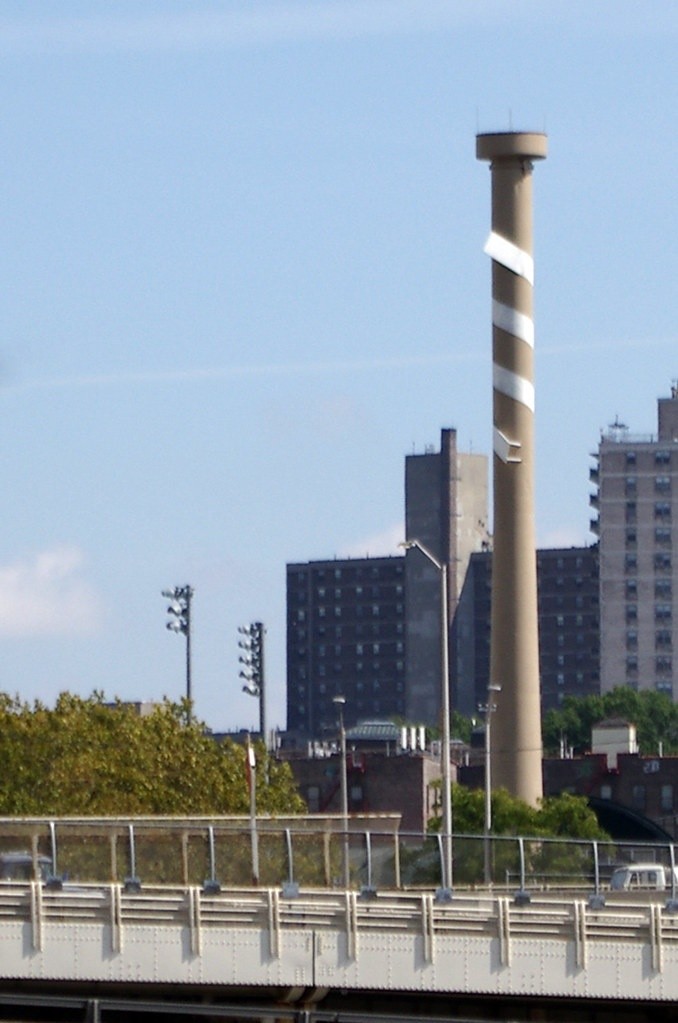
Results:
[0,849,50,883]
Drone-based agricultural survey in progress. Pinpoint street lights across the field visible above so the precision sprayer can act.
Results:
[474,685,502,884]
[398,540,453,892]
[332,697,351,890]
[239,622,267,745]
[162,585,194,725]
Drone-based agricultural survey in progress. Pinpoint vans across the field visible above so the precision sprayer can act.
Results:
[611,863,671,894]
